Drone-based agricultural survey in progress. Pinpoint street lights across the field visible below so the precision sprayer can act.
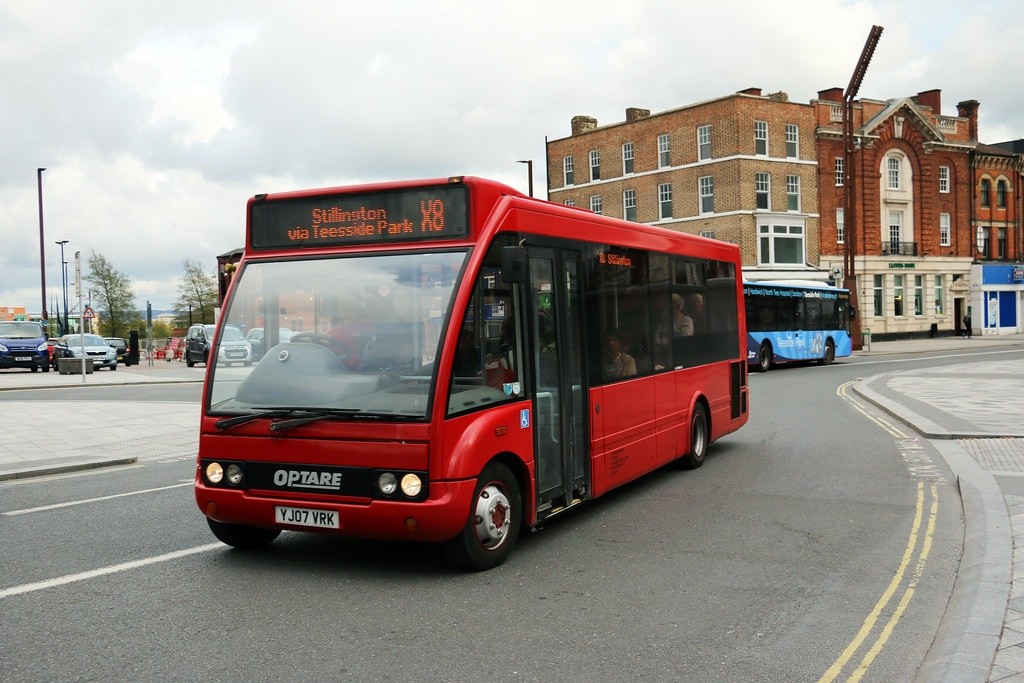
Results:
[37,167,51,338]
[518,159,533,199]
[63,260,69,333]
[54,239,69,335]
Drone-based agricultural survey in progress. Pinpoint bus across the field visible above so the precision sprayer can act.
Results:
[193,175,746,571]
[706,278,856,373]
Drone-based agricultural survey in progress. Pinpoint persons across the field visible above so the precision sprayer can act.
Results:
[328,290,375,373]
[962,312,971,339]
[654,293,693,372]
[606,329,637,380]
[685,293,710,334]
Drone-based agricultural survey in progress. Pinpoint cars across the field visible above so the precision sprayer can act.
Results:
[0,320,51,373]
[246,327,297,362]
[101,338,132,367]
[52,334,117,371]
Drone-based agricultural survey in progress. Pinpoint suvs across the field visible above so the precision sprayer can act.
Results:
[186,323,252,368]
